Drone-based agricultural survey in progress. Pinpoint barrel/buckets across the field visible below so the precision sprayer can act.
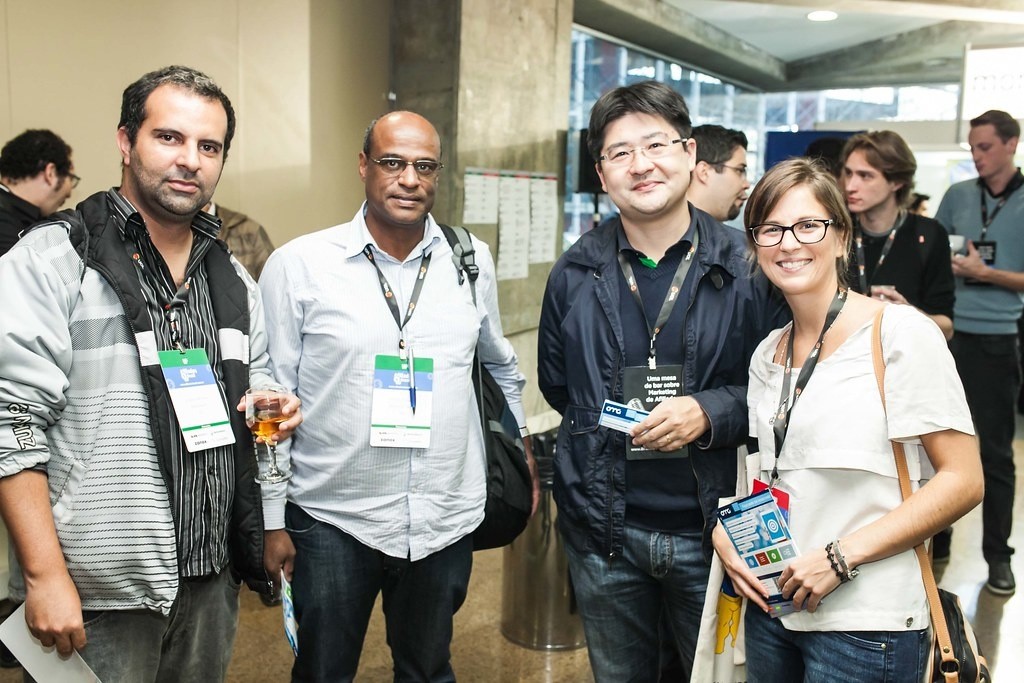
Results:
[500,456,587,650]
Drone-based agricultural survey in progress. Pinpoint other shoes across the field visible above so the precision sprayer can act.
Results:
[258,580,284,606]
[927,526,953,563]
[986,555,1015,597]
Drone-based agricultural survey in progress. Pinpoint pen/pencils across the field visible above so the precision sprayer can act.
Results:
[408,346,416,417]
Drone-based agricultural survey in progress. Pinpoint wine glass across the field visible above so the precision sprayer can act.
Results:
[246,385,295,484]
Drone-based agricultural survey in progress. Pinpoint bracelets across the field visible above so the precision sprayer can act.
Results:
[825,540,859,584]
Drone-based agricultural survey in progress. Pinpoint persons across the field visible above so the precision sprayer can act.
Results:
[0,128,81,259]
[196,197,276,282]
[538,80,792,683]
[711,152,986,683]
[836,131,958,343]
[258,111,538,683]
[903,190,930,219]
[927,109,1024,596]
[0,66,304,683]
[685,122,750,222]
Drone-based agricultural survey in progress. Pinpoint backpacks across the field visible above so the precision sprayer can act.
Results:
[438,223,535,551]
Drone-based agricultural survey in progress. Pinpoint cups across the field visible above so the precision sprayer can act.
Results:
[870,285,896,304]
[947,234,968,258]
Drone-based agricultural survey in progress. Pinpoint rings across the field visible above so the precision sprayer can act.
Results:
[666,434,671,442]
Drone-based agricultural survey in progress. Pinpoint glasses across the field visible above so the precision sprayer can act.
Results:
[55,169,81,189]
[366,154,444,177]
[708,161,746,177]
[598,138,683,163]
[748,219,837,248]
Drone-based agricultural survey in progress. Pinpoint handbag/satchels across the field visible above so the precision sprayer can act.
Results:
[933,586,991,683]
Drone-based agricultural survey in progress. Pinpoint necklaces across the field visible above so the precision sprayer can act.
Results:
[776,287,849,366]
